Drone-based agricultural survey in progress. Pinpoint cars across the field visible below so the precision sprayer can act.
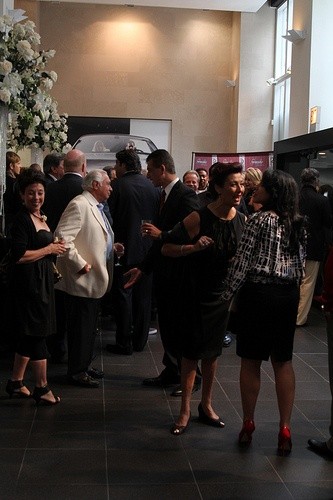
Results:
[69,133,158,174]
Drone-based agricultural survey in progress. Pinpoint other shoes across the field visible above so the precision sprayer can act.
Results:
[131,326,158,334]
[224,331,233,347]
[131,334,144,352]
[107,344,132,355]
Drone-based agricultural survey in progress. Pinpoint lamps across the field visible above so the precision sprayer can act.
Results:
[225,80,235,88]
[7,9,27,22]
[281,29,306,45]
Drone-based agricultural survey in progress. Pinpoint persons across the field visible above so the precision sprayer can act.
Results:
[294,167,333,326]
[216,169,309,451]
[162,162,248,435]
[308,233,333,461]
[0,149,263,406]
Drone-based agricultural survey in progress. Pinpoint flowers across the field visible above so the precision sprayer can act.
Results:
[0,15,70,154]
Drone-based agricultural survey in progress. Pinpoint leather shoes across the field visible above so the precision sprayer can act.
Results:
[308,438,333,460]
[87,368,104,376]
[171,409,192,435]
[198,402,226,427]
[171,382,197,395]
[142,377,180,388]
[70,371,100,386]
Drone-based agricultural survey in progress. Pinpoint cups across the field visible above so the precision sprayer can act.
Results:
[141,220,151,237]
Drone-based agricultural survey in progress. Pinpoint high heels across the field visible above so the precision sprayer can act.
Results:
[33,385,64,406]
[243,419,256,443]
[6,379,33,398]
[279,425,292,444]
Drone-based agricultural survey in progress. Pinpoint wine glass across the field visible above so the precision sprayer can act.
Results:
[53,231,65,258]
[113,243,123,266]
